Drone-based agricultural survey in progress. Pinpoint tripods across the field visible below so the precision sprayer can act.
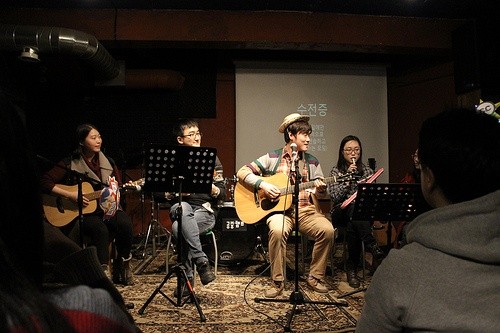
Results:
[254,159,348,332]
[134,143,217,322]
[336,222,370,299]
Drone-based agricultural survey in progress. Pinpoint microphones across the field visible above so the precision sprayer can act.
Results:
[290,142,298,161]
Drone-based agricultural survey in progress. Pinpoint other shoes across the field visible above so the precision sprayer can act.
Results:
[174,279,194,298]
[307,278,328,293]
[196,262,216,285]
[265,283,284,298]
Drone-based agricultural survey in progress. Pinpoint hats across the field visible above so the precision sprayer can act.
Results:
[279,112,311,133]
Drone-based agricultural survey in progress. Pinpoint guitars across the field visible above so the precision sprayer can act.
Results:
[41,177,146,228]
[231,173,340,225]
[367,157,398,248]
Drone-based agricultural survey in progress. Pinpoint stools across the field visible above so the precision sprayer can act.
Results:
[289,231,307,278]
[330,229,367,283]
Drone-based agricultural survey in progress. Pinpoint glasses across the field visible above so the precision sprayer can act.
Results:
[411,149,423,170]
[344,148,360,155]
[183,132,204,137]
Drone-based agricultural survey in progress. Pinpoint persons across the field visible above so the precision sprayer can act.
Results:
[0,149,136,333]
[41,125,134,285]
[237,113,335,298]
[329,135,386,287]
[355,108,500,333]
[165,118,224,296]
